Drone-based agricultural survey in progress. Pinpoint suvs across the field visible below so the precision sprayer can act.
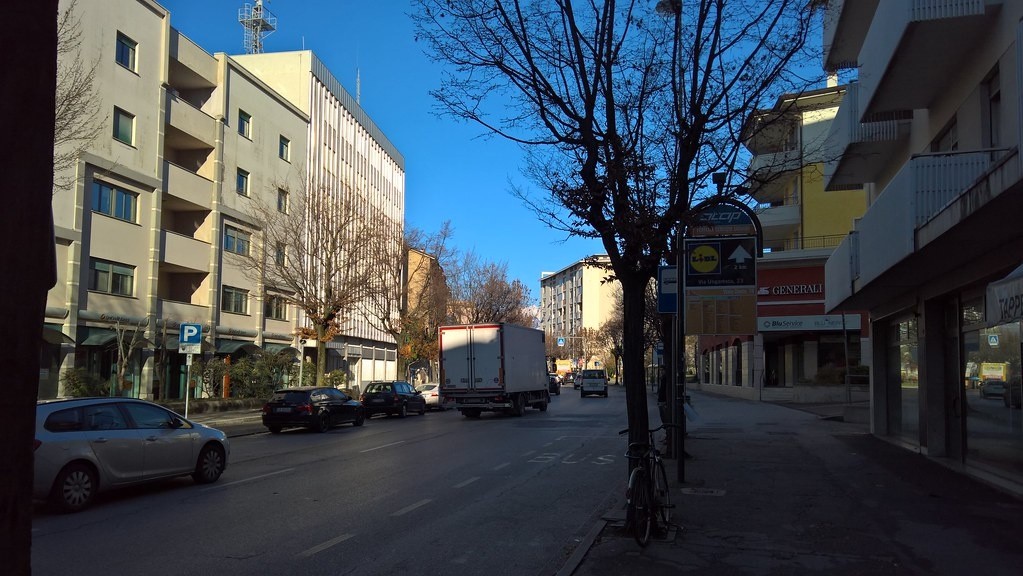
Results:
[979,378,1007,397]
[361,380,426,418]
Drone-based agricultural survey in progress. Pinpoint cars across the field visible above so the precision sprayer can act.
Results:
[416,383,440,412]
[560,373,575,385]
[573,373,581,389]
[262,386,365,432]
[1004,376,1023,407]
[548,373,560,394]
[32,398,230,512]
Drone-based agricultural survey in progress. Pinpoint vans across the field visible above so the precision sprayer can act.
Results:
[580,369,610,398]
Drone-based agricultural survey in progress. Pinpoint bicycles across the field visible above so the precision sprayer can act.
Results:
[617,423,678,544]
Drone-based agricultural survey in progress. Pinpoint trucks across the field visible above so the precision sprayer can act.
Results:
[438,321,557,416]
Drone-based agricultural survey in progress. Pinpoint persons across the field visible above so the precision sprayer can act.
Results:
[657,365,667,443]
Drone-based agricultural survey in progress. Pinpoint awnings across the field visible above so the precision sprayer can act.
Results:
[218,341,261,354]
[44,325,75,346]
[160,337,213,351]
[80,332,158,349]
[265,345,301,357]
